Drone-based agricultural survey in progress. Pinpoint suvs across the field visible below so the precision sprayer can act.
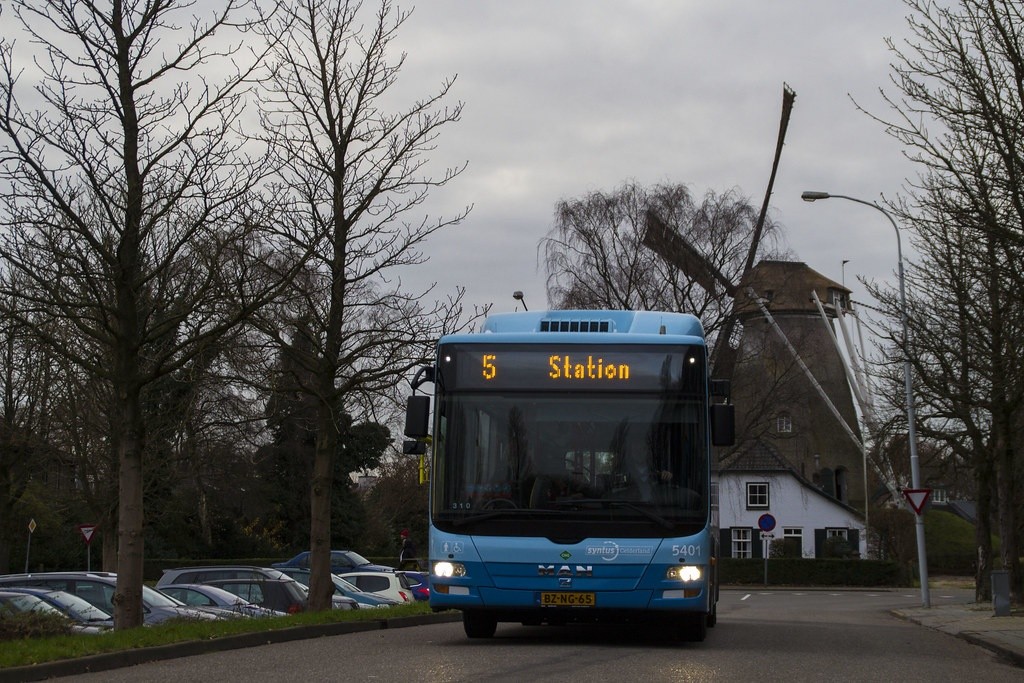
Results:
[275,568,404,611]
[0,572,218,630]
[156,566,360,613]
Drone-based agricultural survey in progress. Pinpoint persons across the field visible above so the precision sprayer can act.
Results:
[398,529,417,570]
[624,434,674,484]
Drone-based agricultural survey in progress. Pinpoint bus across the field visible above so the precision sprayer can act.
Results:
[402,309,734,643]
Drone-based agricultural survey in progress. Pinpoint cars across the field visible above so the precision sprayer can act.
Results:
[0,591,107,638]
[395,570,430,602]
[273,549,395,575]
[159,584,291,621]
[0,587,115,631]
[340,572,417,606]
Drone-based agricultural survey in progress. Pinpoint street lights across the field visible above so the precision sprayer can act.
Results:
[801,189,931,609]
